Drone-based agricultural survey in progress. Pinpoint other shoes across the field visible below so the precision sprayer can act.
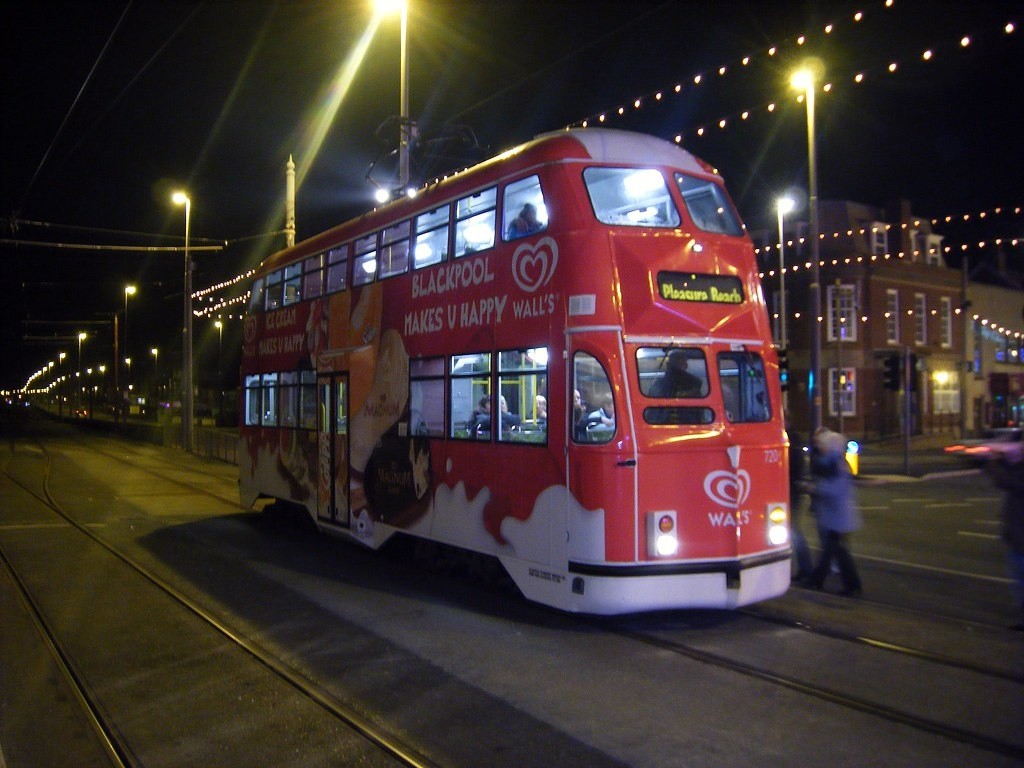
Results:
[799,577,825,590]
[838,584,860,594]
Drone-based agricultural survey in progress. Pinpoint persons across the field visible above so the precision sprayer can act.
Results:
[645,348,705,424]
[969,428,1024,630]
[507,203,543,240]
[468,386,615,443]
[783,410,863,597]
[706,384,734,423]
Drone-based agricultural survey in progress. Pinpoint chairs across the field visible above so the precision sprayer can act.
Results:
[459,422,617,442]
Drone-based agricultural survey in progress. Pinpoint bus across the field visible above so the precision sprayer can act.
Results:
[237,115,795,618]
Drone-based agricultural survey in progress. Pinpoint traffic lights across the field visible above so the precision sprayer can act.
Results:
[908,351,920,393]
[882,353,901,392]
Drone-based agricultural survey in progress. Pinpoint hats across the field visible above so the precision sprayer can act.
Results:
[818,432,846,451]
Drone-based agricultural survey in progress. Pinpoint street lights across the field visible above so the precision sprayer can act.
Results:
[371,0,412,196]
[75,332,87,420]
[169,190,191,449]
[213,320,225,428]
[43,366,47,398]
[124,356,133,404]
[771,191,795,417]
[122,283,137,422]
[58,351,67,417]
[788,64,823,477]
[150,348,161,423]
[48,361,54,414]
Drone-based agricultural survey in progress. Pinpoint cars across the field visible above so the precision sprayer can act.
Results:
[943,426,1024,469]
